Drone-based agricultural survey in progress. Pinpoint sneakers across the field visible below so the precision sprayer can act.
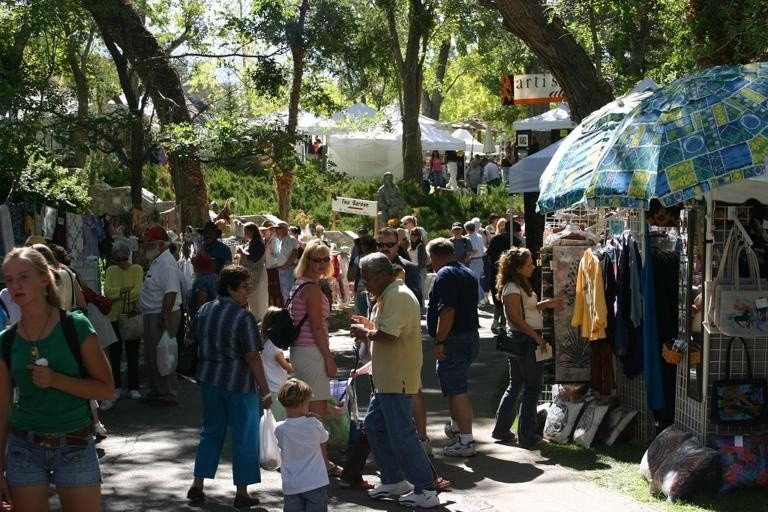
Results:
[129,390,141,399]
[421,438,431,455]
[445,425,459,438]
[116,388,120,399]
[94,421,106,433]
[444,437,473,456]
[368,481,414,500]
[398,490,439,507]
[100,391,115,410]
[188,487,205,497]
[235,495,259,507]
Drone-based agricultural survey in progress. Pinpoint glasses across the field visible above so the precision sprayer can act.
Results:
[377,242,397,247]
[115,256,128,261]
[404,223,410,225]
[309,256,330,262]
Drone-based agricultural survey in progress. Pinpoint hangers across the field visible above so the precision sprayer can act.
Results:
[579,213,637,267]
[547,215,597,245]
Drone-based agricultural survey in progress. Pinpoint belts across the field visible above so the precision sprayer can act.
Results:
[8,427,87,445]
[471,257,483,259]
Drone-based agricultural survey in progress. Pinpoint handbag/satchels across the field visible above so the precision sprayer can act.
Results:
[702,228,768,336]
[712,336,768,422]
[497,281,525,354]
[62,269,74,304]
[118,291,144,340]
[471,218,480,227]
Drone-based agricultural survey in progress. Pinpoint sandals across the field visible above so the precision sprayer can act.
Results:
[328,462,343,475]
[434,477,451,489]
[337,481,374,488]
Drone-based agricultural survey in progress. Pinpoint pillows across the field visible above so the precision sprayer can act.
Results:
[640,424,690,483]
[573,401,609,449]
[652,439,718,501]
[714,435,768,496]
[543,397,583,444]
[598,404,638,446]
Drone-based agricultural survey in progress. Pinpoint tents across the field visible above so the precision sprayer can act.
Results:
[440,114,501,148]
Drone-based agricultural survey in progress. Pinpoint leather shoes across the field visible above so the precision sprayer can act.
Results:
[138,390,157,401]
[148,399,178,406]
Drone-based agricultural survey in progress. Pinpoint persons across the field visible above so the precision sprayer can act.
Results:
[0,248,113,512]
[472,218,491,252]
[258,306,291,422]
[183,263,283,508]
[492,249,567,447]
[262,240,347,480]
[429,150,445,188]
[179,240,195,301]
[276,378,330,511]
[349,253,439,508]
[288,224,306,263]
[356,261,436,463]
[446,150,460,189]
[278,221,298,306]
[168,242,182,266]
[106,241,142,401]
[466,153,504,190]
[463,222,490,310]
[406,228,426,315]
[30,244,110,436]
[481,210,528,242]
[377,171,405,227]
[179,251,219,383]
[235,221,268,333]
[357,228,423,360]
[52,245,121,410]
[259,217,281,308]
[446,223,474,268]
[339,359,452,489]
[197,222,232,278]
[384,217,427,253]
[347,232,378,316]
[137,226,182,407]
[481,220,526,333]
[422,237,484,456]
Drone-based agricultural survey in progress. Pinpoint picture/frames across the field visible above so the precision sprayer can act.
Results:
[517,129,530,148]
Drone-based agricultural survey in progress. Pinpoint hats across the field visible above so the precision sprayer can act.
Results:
[452,222,462,229]
[197,222,222,231]
[145,225,169,240]
[191,252,216,272]
[271,223,288,227]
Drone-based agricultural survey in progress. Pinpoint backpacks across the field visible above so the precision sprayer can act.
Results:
[264,282,317,350]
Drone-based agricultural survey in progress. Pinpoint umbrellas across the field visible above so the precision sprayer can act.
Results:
[250,108,335,163]
[328,106,467,172]
[533,60,768,446]
[516,104,580,130]
[511,138,558,191]
[340,104,379,119]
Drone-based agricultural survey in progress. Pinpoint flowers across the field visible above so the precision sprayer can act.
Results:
[295,210,313,230]
